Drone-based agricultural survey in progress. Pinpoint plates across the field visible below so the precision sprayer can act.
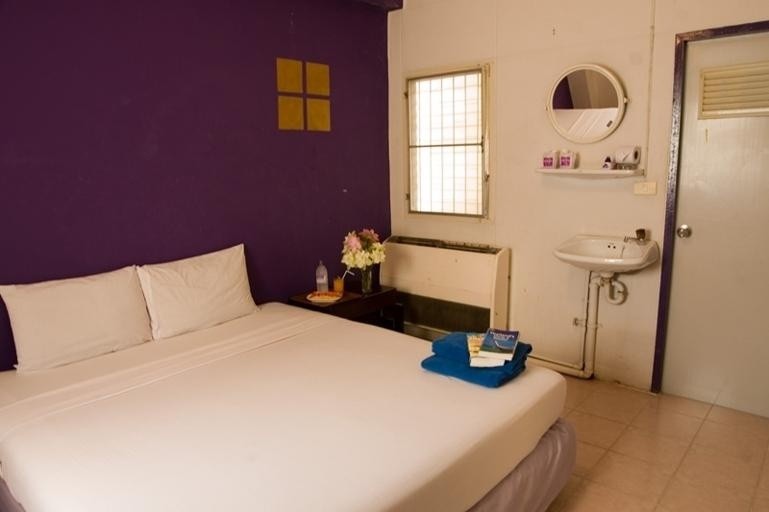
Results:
[305,291,343,303]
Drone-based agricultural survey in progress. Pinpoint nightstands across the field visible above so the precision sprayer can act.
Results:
[289,285,399,331]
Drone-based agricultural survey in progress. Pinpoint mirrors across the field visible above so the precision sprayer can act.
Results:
[546,64,628,144]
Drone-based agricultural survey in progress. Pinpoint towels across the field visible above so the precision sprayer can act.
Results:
[420,332,532,388]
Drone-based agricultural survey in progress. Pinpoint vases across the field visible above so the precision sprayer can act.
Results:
[360,266,374,293]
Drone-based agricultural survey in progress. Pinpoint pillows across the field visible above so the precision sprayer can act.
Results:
[0,242,259,371]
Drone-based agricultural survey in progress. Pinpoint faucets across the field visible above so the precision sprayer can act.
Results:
[624,229,646,245]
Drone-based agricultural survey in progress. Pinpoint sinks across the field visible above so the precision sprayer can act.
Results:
[553,234,658,274]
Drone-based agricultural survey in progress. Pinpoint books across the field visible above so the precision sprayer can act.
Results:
[466,328,519,368]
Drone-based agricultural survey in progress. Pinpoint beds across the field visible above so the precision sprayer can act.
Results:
[1,301,580,512]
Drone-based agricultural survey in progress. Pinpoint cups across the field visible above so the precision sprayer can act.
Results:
[332,276,344,292]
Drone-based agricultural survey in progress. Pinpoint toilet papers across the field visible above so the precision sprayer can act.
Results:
[614,145,641,164]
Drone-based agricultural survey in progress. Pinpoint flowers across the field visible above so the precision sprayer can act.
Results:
[339,228,386,292]
